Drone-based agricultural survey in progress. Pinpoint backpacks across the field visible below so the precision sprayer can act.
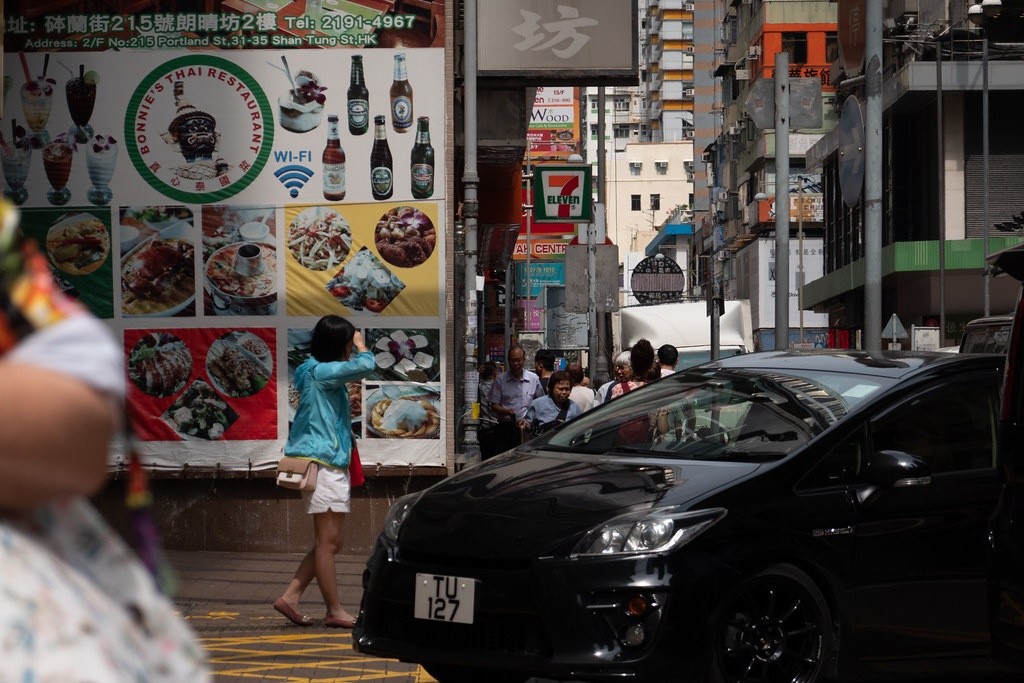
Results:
[611,379,650,446]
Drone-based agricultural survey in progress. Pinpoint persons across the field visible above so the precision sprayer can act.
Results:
[0,202,210,683]
[865,412,949,482]
[273,313,376,625]
[478,339,696,461]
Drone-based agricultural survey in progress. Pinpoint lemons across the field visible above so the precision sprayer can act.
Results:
[84,70,100,85]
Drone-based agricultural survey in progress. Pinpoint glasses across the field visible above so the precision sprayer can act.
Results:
[617,366,631,370]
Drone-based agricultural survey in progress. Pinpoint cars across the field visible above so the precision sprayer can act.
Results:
[352,348,1024,683]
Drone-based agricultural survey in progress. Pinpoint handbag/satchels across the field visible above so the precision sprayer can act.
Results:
[0,494,211,683]
[276,457,319,491]
[349,448,365,487]
[532,420,561,439]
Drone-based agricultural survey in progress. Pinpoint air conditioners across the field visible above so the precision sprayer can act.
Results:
[624,98,630,102]
[686,89,695,96]
[687,46,695,56]
[686,4,694,12]
[634,163,641,168]
[687,131,695,140]
[613,125,620,129]
[661,163,667,167]
[688,161,693,166]
[687,174,695,183]
[729,120,745,135]
[701,154,713,163]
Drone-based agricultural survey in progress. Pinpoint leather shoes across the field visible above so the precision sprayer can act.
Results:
[273,595,314,626]
[325,613,357,628]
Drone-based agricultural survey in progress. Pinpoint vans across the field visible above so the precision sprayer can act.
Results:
[959,313,1014,357]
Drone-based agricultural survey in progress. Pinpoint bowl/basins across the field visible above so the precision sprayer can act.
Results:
[119,225,141,257]
[239,222,270,242]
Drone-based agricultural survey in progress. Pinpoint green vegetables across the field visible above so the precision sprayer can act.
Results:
[128,336,268,440]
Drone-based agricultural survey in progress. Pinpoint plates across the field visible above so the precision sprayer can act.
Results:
[237,333,269,357]
[121,218,195,318]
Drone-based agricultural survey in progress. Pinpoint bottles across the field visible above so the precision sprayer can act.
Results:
[348,55,369,135]
[371,116,393,200]
[390,53,414,133]
[411,117,434,199]
[322,115,346,201]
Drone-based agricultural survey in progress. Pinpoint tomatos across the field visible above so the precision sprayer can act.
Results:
[332,286,351,297]
[365,298,386,312]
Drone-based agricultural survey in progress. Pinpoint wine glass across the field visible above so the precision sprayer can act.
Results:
[66,77,96,144]
[42,143,73,205]
[85,138,119,206]
[0,141,32,205]
[21,80,52,149]
